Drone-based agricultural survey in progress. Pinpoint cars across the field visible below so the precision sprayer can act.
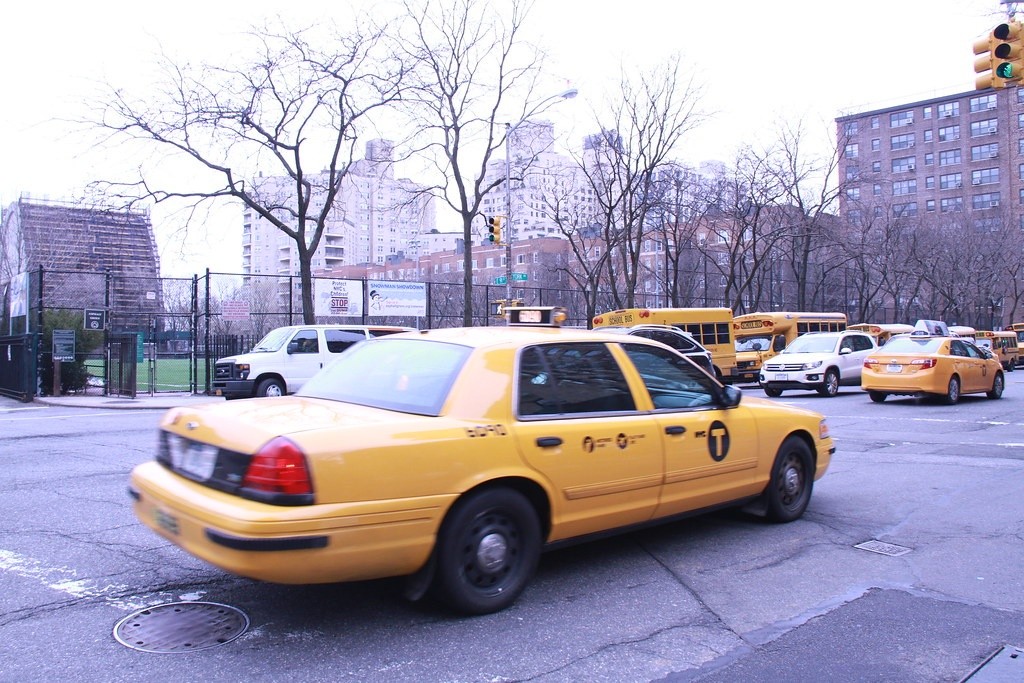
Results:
[130,326,835,615]
[862,320,1004,404]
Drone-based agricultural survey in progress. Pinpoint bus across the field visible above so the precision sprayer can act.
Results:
[847,324,1019,372]
[732,311,846,384]
[593,306,739,384]
[1005,323,1024,366]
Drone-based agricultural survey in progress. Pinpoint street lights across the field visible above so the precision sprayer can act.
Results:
[506,90,581,304]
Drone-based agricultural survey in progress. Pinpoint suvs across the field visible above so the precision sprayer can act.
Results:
[760,329,878,397]
[596,323,716,378]
[212,325,369,395]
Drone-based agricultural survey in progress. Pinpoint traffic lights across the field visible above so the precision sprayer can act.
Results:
[490,300,506,319]
[974,21,1024,89]
[490,218,500,242]
